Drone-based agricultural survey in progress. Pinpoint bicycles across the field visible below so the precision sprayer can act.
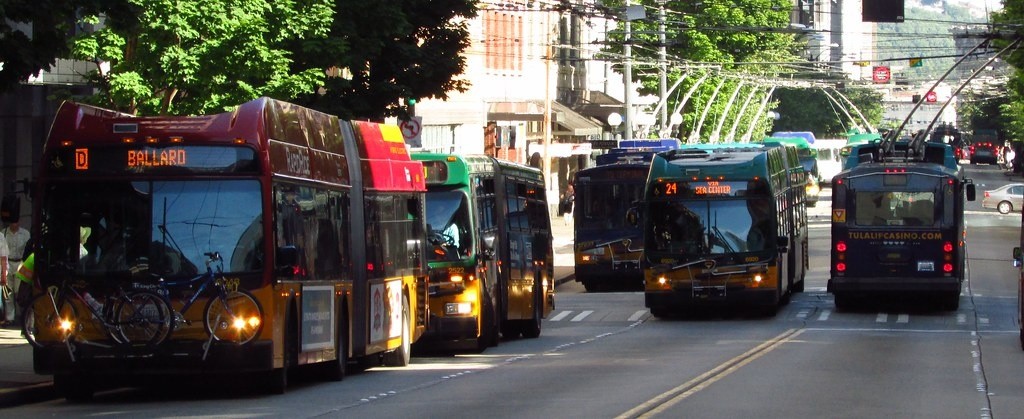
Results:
[22,252,263,351]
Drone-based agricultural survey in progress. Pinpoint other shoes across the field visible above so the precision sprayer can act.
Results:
[0,320,14,327]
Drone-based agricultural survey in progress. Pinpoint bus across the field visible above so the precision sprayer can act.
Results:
[763,131,820,207]
[406,154,555,354]
[845,140,958,172]
[924,125,961,164]
[625,142,809,314]
[558,151,666,292]
[827,163,976,311]
[22,97,430,399]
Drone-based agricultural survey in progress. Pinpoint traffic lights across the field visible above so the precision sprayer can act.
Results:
[405,98,416,117]
[927,92,937,102]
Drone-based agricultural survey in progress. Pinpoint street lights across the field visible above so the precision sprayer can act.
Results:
[607,112,622,140]
[671,113,683,138]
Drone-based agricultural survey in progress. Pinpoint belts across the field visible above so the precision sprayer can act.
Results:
[8,258,21,262]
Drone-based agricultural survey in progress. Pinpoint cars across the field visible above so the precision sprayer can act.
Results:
[981,183,1024,214]
[816,140,847,183]
[970,143,997,165]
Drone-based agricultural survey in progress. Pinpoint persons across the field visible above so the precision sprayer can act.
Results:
[1001,139,1014,170]
[67,227,92,258]
[0,214,35,338]
[559,182,575,217]
